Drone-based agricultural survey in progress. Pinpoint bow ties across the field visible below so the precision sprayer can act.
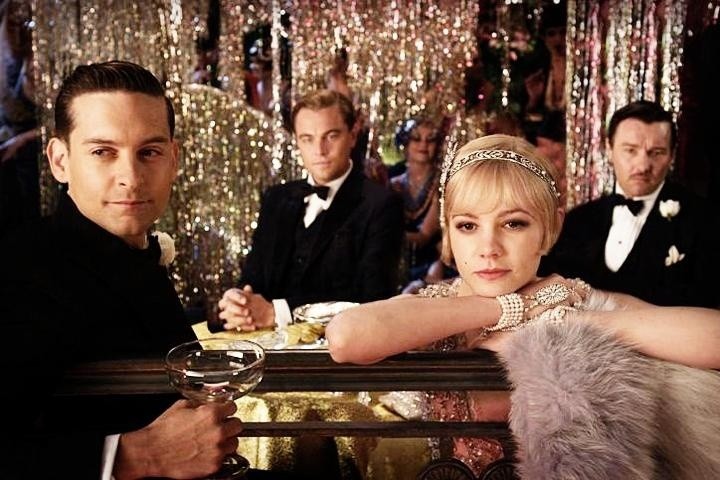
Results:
[302,182,329,200]
[609,193,644,216]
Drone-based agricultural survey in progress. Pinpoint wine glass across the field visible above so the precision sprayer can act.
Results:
[165,337,264,478]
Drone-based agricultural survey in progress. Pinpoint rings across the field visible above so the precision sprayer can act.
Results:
[234,326,244,333]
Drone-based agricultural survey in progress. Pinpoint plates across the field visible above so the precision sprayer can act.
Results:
[292,300,360,327]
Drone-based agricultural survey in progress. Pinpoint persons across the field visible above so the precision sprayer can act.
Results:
[0,58,246,480]
[202,86,408,341]
[321,130,720,480]
[1,0,720,297]
[532,99,719,312]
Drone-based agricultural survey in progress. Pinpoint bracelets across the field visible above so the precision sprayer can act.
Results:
[483,293,527,332]
